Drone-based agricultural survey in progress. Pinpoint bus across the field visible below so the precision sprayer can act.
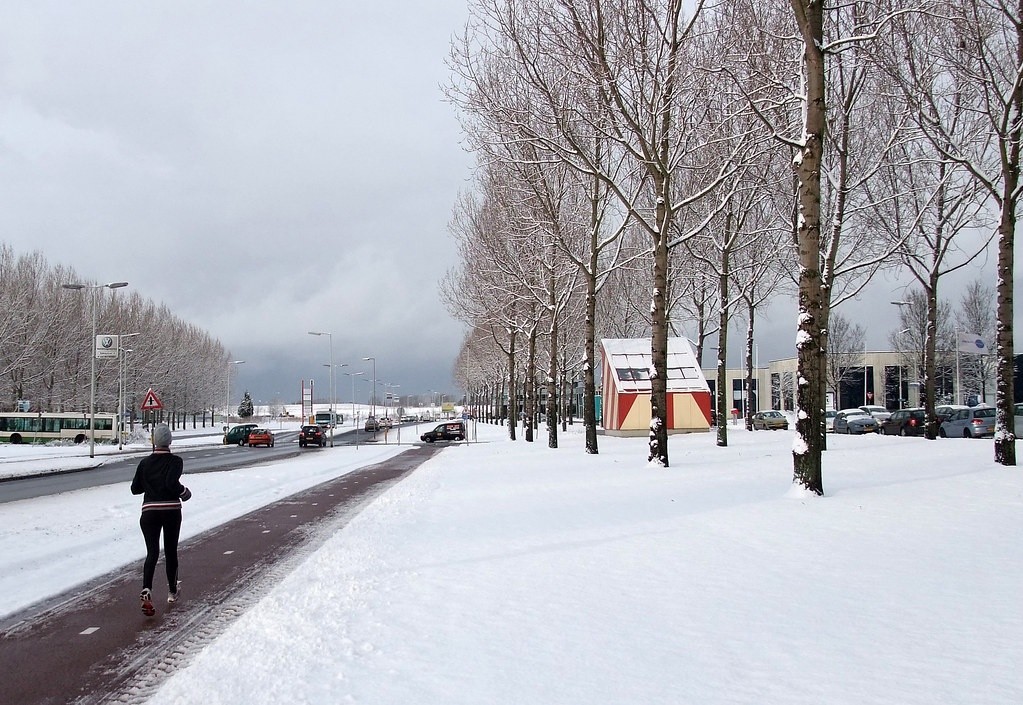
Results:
[0,411,125,444]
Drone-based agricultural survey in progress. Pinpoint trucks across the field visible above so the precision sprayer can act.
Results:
[315,411,343,428]
[423,414,430,421]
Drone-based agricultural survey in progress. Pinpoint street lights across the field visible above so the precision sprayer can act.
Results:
[119,333,141,450]
[323,356,376,439]
[890,301,917,407]
[62,282,128,458]
[227,360,245,435]
[466,343,471,445]
[364,380,400,419]
[307,332,334,447]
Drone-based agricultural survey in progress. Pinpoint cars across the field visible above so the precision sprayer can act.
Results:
[401,415,419,423]
[825,404,1023,441]
[754,411,788,431]
[249,429,275,447]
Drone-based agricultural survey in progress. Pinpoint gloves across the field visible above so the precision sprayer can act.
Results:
[180,487,192,502]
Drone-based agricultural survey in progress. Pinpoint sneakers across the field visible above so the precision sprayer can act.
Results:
[140,588,155,617]
[167,580,181,604]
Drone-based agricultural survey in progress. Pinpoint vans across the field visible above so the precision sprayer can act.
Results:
[223,425,258,446]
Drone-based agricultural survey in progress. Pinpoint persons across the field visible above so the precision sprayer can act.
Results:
[131,423,192,616]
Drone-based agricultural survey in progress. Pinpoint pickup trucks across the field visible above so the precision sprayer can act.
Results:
[379,418,393,429]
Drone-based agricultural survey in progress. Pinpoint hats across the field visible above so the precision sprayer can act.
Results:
[154,423,171,446]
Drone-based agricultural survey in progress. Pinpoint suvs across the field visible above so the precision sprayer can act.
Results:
[365,420,380,432]
[420,423,465,443]
[298,424,327,448]
